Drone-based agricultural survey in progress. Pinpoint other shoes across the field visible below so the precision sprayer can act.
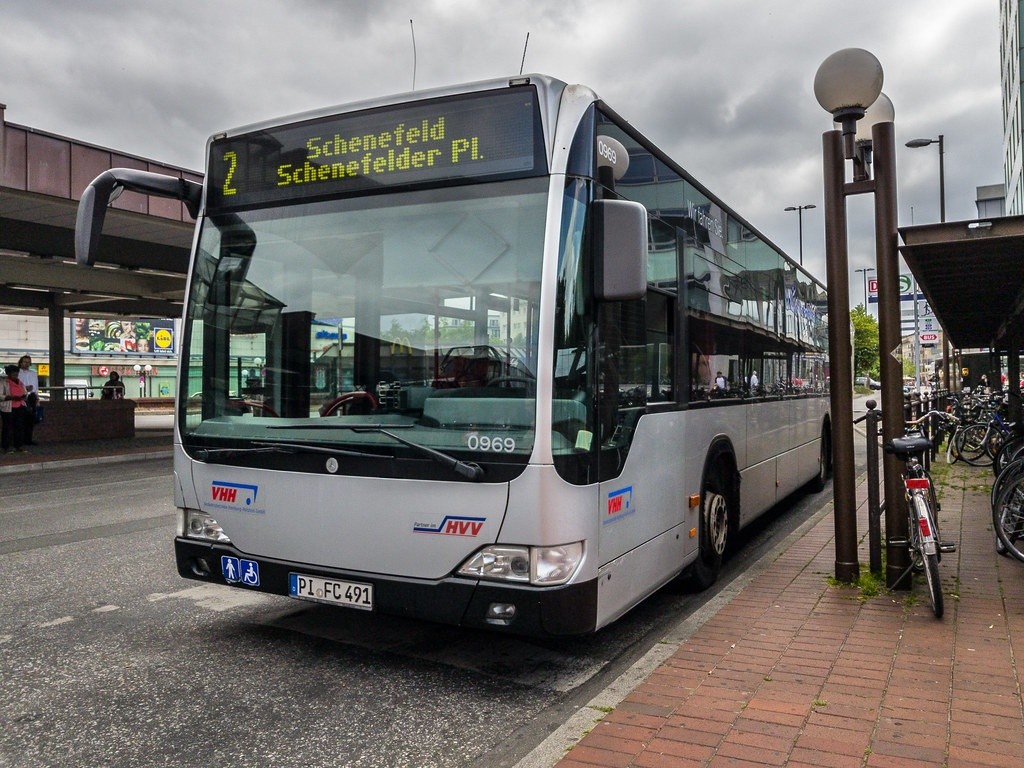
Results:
[17,447,28,452]
[2,448,14,453]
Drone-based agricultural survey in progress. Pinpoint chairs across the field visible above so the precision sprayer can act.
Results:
[431,345,503,390]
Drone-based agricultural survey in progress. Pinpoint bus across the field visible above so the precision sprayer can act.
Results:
[72,29,853,644]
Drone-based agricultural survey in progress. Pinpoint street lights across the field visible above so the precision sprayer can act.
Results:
[134,365,152,398]
[855,267,875,317]
[813,46,914,594]
[784,204,816,266]
[904,133,949,408]
[242,358,266,389]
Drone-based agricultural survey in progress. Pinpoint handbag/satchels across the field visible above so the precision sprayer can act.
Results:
[34,399,44,421]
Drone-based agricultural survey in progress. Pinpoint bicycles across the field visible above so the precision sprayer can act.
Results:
[944,384,1024,564]
[854,410,960,622]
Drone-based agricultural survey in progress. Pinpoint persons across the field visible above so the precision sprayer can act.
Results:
[101,371,125,400]
[750,371,759,389]
[714,371,727,389]
[0,355,41,454]
